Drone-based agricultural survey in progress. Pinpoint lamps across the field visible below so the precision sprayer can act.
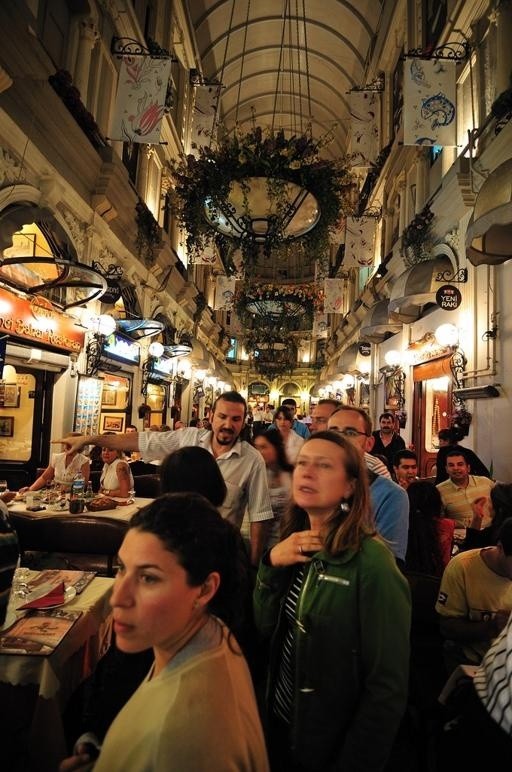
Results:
[435,323,467,407]
[81,310,117,376]
[385,350,406,409]
[142,342,164,398]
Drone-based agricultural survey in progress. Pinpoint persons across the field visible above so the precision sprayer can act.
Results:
[0,391,512,772]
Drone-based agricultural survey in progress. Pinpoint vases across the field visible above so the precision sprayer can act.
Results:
[202,176,322,244]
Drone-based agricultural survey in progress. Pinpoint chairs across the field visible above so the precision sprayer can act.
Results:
[36,468,161,497]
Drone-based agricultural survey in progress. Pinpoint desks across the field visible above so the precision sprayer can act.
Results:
[6,492,157,577]
[0,570,116,727]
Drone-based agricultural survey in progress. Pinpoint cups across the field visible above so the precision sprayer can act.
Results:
[0,480,7,495]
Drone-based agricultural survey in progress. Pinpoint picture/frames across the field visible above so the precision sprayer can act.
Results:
[101,389,116,405]
[0,383,21,408]
[0,416,14,437]
[1,233,36,258]
[103,416,123,432]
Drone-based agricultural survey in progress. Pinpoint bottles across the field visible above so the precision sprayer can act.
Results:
[73,472,85,499]
[26,493,33,507]
[86,481,93,498]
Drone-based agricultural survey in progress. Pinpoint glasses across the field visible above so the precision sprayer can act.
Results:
[328,426,359,437]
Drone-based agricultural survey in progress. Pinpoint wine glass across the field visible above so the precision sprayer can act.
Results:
[47,480,55,489]
[127,491,136,506]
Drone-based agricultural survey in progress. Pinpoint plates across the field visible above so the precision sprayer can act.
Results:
[26,585,76,610]
[42,496,62,503]
[14,495,24,500]
[0,610,16,631]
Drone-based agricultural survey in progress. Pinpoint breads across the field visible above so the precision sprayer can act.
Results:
[90,497,115,509]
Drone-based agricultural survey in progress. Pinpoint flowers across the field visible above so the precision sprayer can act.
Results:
[160,104,369,273]
[222,274,327,382]
